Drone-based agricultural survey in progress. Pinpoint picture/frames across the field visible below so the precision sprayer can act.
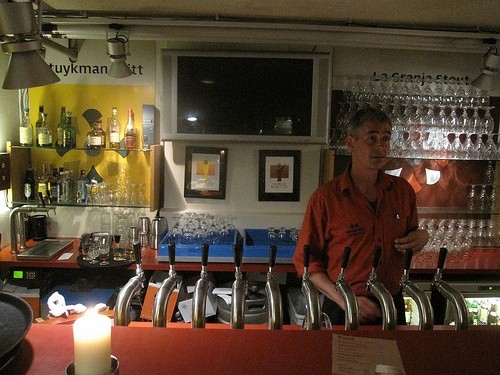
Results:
[258,150,302,203]
[183,145,227,199]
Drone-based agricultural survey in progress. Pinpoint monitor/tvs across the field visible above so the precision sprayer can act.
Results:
[159,48,332,145]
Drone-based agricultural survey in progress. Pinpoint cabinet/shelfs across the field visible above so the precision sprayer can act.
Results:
[1,237,321,326]
[5,139,164,211]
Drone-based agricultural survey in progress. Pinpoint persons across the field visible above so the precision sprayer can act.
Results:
[291,106,430,325]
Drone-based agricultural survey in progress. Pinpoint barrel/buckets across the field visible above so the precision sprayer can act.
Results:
[217,281,271,325]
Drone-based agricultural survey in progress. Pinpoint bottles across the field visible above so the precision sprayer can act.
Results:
[108,107,121,148]
[23,163,89,204]
[36,106,49,147]
[466,299,497,326]
[86,121,101,148]
[124,109,137,148]
[96,119,107,148]
[56,107,71,148]
[44,113,52,147]
[66,112,76,148]
[19,109,33,146]
[404,299,411,326]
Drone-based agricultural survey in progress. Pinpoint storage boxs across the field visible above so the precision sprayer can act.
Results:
[2,285,41,320]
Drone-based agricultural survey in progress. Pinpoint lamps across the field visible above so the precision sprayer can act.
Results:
[469,46,500,92]
[105,30,132,79]
[1,0,72,89]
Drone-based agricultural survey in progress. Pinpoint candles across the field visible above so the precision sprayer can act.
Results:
[71,314,112,375]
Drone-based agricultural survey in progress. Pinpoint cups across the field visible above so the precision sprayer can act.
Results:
[81,211,168,261]
[24,215,31,241]
[31,214,48,241]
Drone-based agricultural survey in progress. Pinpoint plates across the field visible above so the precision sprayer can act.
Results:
[0,291,34,372]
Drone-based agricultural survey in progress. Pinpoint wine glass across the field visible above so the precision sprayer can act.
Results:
[465,162,500,212]
[169,211,236,246]
[88,244,111,265]
[416,216,500,256]
[85,181,146,206]
[331,73,499,162]
[267,224,300,245]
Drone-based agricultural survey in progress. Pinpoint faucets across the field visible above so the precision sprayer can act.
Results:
[334,247,359,331]
[9,206,32,254]
[301,244,321,330]
[190,243,212,328]
[264,244,283,330]
[398,247,434,330]
[365,246,397,330]
[152,242,178,327]
[429,247,468,331]
[113,240,146,326]
[230,244,248,329]
